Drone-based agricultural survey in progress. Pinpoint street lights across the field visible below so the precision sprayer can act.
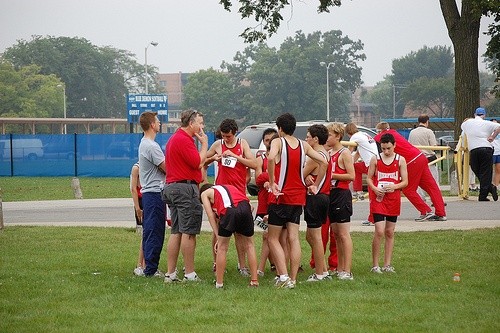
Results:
[144,40,157,95]
[319,62,336,122]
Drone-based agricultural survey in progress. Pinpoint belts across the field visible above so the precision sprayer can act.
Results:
[180,180,197,184]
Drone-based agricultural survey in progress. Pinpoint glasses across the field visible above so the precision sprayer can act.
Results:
[187,109,197,121]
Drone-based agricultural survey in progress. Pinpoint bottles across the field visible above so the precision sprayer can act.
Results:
[159,181,164,192]
[376,187,385,202]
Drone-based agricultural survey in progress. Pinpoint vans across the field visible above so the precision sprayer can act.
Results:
[236,121,379,196]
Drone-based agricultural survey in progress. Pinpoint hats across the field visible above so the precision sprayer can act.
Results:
[476,108,486,115]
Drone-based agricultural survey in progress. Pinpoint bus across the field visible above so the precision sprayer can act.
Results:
[379,118,500,154]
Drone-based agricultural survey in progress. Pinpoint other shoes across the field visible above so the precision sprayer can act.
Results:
[300,272,332,284]
[480,198,490,201]
[491,186,498,201]
[256,269,265,277]
[272,275,296,289]
[352,193,364,200]
[335,270,353,280]
[362,220,375,226]
[134,263,259,289]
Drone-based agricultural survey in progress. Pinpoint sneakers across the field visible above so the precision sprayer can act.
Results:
[428,215,447,221]
[381,265,396,273]
[416,211,435,222]
[371,266,382,274]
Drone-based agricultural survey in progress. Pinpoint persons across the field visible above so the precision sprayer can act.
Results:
[408,115,447,206]
[200,114,356,288]
[368,134,408,275]
[368,122,447,222]
[129,162,143,230]
[134,112,167,277]
[345,123,381,227]
[454,108,500,202]
[164,108,208,281]
